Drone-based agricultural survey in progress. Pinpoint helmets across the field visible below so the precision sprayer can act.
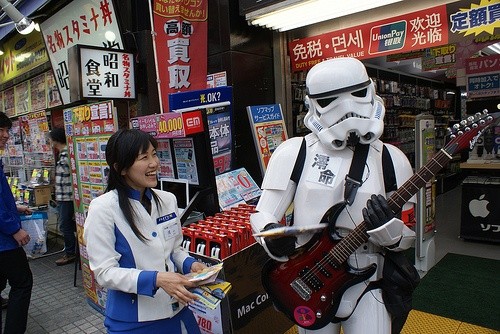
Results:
[303,57,385,150]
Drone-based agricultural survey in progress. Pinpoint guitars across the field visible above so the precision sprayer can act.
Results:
[262,109,494,330]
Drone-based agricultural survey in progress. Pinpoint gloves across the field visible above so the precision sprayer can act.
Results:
[262,223,295,258]
[362,194,403,247]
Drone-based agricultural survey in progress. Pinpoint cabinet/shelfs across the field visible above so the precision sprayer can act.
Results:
[435,157,460,196]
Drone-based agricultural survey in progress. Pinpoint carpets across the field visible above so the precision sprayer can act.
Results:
[412,253,500,331]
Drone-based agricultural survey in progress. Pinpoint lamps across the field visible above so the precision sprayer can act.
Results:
[245,0,402,33]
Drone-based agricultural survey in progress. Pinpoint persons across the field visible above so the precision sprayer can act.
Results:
[250,57,418,334]
[83,128,207,334]
[49,127,77,265]
[0,112,33,334]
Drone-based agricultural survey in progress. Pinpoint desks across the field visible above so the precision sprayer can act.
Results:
[21,184,51,204]
[19,212,49,257]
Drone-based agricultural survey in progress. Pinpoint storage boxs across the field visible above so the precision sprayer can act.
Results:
[188,241,273,334]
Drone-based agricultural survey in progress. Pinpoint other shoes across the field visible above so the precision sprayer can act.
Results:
[56,255,75,265]
[0,298,8,309]
